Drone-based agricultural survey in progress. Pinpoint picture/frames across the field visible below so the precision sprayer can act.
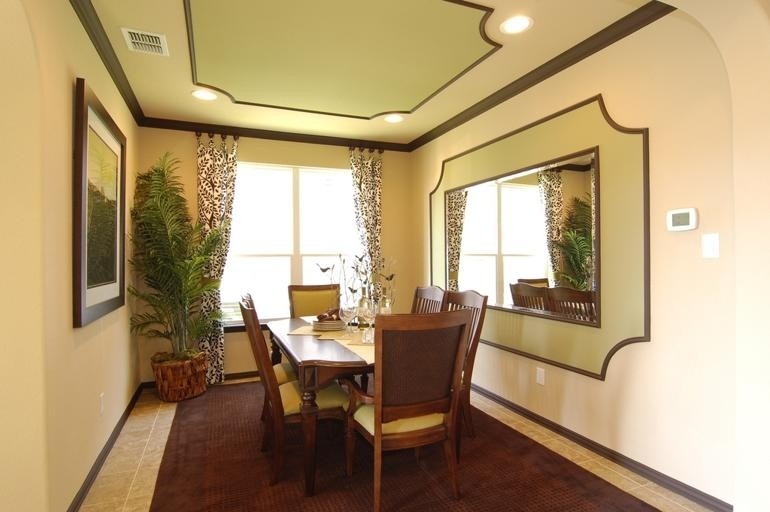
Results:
[71,75,126,330]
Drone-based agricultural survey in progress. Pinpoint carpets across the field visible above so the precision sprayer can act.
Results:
[150,379,662,511]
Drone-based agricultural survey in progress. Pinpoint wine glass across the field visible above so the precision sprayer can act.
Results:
[338,303,392,343]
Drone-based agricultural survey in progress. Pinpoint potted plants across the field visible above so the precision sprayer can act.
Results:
[127,151,228,402]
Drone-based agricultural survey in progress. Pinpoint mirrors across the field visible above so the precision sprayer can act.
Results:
[429,94,652,381]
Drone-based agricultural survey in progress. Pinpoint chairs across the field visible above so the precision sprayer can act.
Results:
[411,286,444,312]
[441,289,488,467]
[288,284,341,318]
[337,309,472,511]
[239,301,360,487]
[508,279,597,323]
[243,292,312,454]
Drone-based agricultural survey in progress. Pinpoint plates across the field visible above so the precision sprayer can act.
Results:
[313,320,345,332]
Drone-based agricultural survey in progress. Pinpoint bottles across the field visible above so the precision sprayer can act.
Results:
[358,287,372,330]
[380,287,391,313]
